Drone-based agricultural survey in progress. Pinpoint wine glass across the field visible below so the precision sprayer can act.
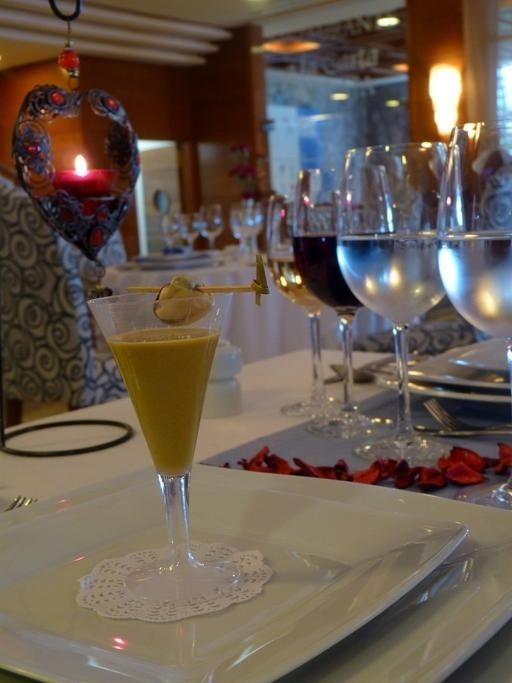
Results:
[336,140,443,455]
[436,123,512,505]
[263,149,376,443]
[85,289,241,603]
[160,199,265,266]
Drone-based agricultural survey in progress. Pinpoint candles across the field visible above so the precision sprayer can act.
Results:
[51,153,114,213]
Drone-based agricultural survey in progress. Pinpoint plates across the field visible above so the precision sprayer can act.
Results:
[0,455,512,683]
[376,335,512,407]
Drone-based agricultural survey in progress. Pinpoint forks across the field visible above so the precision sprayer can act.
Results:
[418,397,511,430]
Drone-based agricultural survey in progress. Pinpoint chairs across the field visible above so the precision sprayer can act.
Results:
[0,173,132,409]
[350,163,512,356]
